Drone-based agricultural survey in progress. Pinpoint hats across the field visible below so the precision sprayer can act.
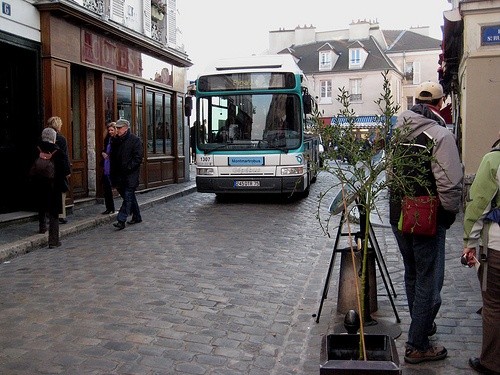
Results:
[416,82,445,100]
[116,119,130,127]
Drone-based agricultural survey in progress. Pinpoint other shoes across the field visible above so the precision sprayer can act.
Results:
[59,218,67,223]
[39,229,47,234]
[49,242,61,248]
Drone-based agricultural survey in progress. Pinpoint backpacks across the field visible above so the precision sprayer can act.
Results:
[29,145,58,191]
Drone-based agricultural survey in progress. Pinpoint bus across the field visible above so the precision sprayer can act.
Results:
[185,54,324,200]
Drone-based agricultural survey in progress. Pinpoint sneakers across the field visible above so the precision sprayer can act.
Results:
[113,221,125,228]
[427,322,436,336]
[127,220,136,225]
[405,342,447,363]
[469,357,500,375]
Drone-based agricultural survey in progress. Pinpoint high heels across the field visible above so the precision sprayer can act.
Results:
[102,206,114,214]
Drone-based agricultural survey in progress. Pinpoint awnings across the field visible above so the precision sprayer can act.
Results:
[329,115,387,129]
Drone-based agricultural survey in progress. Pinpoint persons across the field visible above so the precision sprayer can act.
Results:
[109,119,144,229]
[267,106,299,132]
[190,119,207,165]
[47,117,69,224]
[100,122,132,216]
[389,81,464,364]
[23,127,71,249]
[463,139,500,375]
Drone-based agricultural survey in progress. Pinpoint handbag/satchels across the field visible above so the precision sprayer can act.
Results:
[397,195,440,237]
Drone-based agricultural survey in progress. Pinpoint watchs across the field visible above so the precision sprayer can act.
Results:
[461,253,468,265]
